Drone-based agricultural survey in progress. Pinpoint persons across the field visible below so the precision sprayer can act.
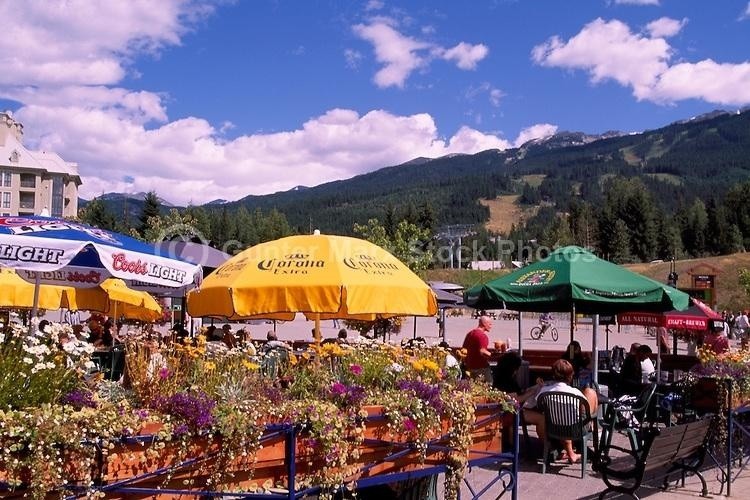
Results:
[721,310,750,343]
[463,316,656,465]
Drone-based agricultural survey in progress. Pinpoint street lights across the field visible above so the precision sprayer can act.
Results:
[446,239,454,268]
[664,255,679,355]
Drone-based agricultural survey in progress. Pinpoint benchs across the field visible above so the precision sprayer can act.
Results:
[591,416,716,500]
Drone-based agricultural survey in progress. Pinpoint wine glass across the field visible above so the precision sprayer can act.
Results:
[495,342,499,353]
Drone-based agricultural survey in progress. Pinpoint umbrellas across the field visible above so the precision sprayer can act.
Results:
[463,244,725,386]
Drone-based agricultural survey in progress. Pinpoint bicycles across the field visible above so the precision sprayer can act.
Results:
[530,317,558,341]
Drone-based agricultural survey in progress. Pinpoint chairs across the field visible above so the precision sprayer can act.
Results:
[536,381,658,480]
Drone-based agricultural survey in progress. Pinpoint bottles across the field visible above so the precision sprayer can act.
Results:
[507,336,512,349]
